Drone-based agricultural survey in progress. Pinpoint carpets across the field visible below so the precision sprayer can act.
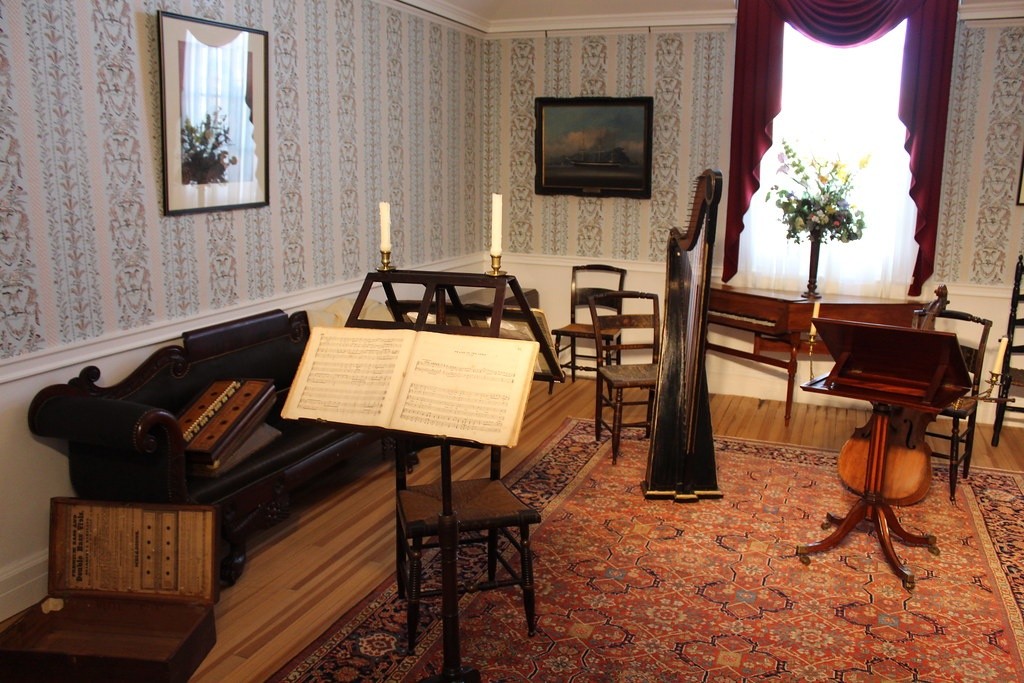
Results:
[265,416,1024,683]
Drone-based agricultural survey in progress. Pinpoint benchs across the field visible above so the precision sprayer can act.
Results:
[28,309,395,586]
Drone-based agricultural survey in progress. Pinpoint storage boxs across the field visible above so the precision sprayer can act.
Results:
[0,497,222,683]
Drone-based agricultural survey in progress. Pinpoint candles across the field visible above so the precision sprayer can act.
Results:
[991,337,1008,374]
[490,193,503,256]
[379,201,391,251]
[810,301,820,335]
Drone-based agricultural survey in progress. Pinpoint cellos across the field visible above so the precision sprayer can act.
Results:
[835,284,949,508]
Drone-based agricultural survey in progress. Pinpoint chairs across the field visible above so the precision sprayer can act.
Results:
[395,439,542,656]
[548,264,627,401]
[912,310,992,501]
[992,254,1024,447]
[588,291,661,467]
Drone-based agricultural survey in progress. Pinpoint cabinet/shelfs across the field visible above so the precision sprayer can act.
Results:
[459,288,539,307]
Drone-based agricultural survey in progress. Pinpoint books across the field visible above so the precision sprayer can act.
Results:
[281,328,539,448]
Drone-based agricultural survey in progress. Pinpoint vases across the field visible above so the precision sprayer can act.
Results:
[801,230,822,298]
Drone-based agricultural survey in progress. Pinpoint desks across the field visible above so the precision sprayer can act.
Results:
[796,373,971,590]
[706,275,931,427]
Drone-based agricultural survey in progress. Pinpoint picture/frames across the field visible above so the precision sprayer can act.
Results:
[1016,145,1024,206]
[157,10,269,216]
[535,96,653,199]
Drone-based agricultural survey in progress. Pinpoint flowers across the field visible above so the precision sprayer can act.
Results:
[765,139,869,244]
